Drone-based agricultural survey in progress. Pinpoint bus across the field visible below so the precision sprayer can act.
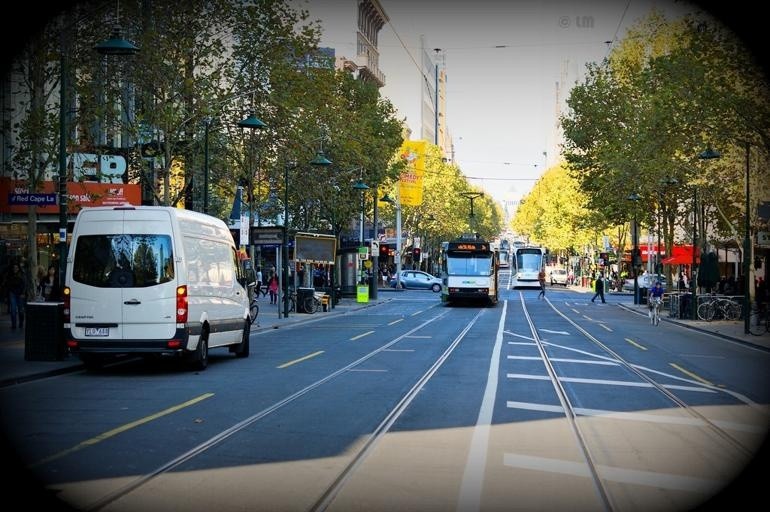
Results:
[437,232,501,310]
[495,236,547,289]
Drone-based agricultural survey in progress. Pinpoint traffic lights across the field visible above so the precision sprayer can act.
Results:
[413,247,421,262]
[600,253,610,267]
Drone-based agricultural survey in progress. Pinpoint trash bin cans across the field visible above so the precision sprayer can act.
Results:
[637,287,648,304]
[23,301,65,361]
[296,288,315,314]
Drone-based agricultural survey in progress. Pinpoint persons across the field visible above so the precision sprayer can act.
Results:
[380,265,390,288]
[591,274,607,304]
[40,264,59,302]
[255,266,265,298]
[537,268,546,299]
[649,282,664,321]
[7,263,27,330]
[264,264,275,295]
[297,264,327,287]
[677,275,687,289]
[268,270,279,305]
[719,275,764,296]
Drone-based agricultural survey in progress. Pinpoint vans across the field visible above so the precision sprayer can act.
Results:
[57,201,256,375]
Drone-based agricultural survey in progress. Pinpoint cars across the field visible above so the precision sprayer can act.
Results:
[389,268,442,294]
[549,268,569,288]
[620,273,680,291]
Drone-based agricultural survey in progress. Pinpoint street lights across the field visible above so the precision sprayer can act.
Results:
[371,182,394,299]
[283,133,334,318]
[201,93,268,215]
[628,190,675,288]
[56,0,141,295]
[304,165,371,228]
[659,160,697,321]
[416,212,436,238]
[698,130,752,336]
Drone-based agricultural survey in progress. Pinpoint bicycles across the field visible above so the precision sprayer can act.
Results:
[282,288,320,315]
[747,309,770,336]
[647,299,664,327]
[696,294,742,322]
[250,297,259,327]
